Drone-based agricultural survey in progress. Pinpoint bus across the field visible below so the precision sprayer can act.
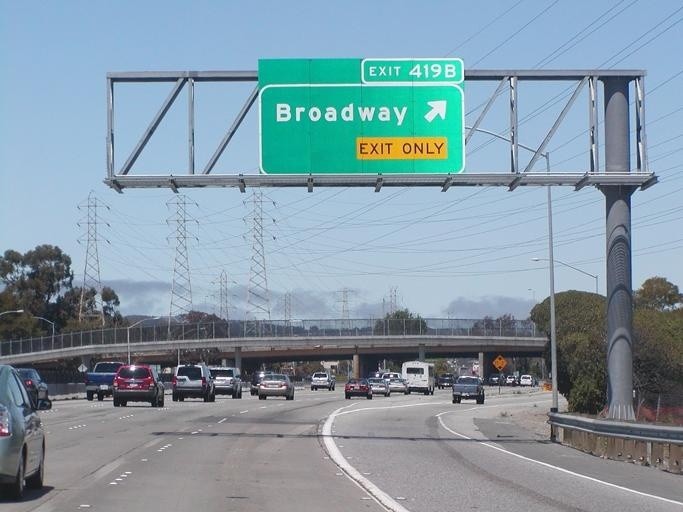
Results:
[401,360,435,396]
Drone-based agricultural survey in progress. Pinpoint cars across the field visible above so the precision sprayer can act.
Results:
[487,373,535,388]
[259,372,295,401]
[0,363,46,493]
[344,377,372,399]
[367,378,390,397]
[13,366,52,410]
[387,378,408,394]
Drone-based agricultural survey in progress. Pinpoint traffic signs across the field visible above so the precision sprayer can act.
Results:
[258,82,465,179]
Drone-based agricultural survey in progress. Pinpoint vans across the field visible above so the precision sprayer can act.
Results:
[250,371,274,396]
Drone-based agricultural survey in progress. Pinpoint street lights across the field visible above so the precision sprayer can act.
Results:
[29,315,55,349]
[0,308,24,320]
[532,258,600,296]
[125,314,161,365]
[528,287,537,337]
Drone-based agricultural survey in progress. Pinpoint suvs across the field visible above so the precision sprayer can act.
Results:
[310,370,335,390]
[172,362,215,402]
[208,367,243,400]
[110,363,163,408]
[380,372,400,380]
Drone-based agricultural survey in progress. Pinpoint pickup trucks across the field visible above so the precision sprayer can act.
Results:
[438,373,456,389]
[452,376,485,403]
[84,360,124,401]
